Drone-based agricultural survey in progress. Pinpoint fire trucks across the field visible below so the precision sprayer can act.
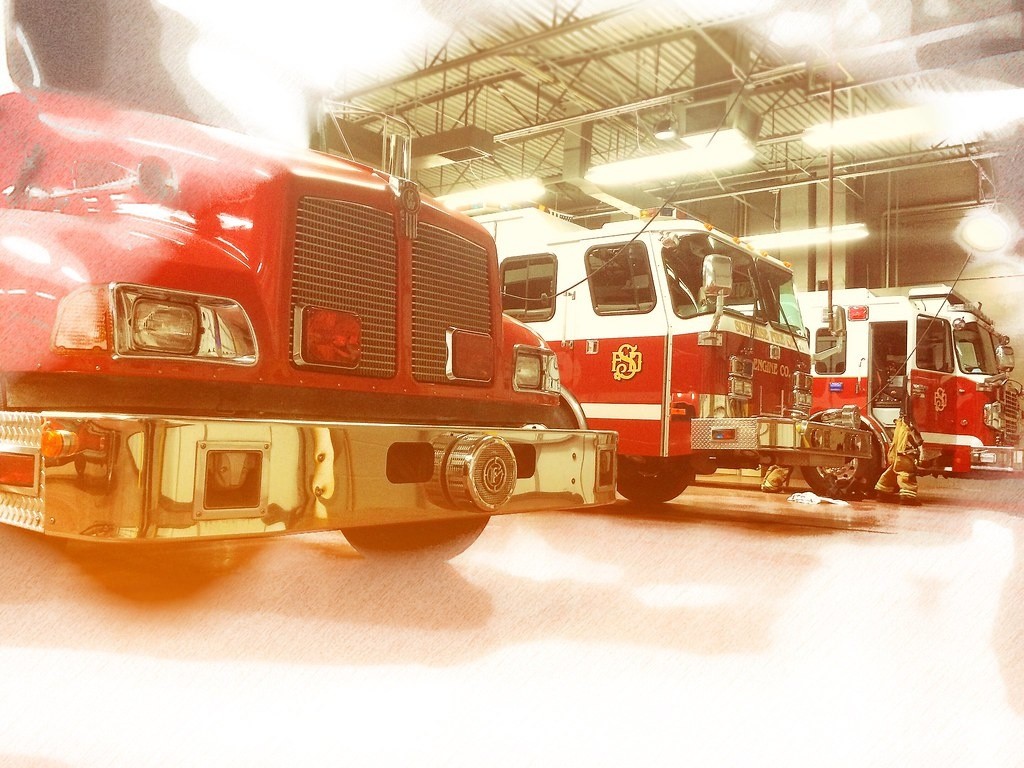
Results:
[731,280,1024,502]
[2,1,619,564]
[438,201,872,507]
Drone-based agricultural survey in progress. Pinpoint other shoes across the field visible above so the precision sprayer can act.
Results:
[899,497,922,506]
[876,491,900,503]
